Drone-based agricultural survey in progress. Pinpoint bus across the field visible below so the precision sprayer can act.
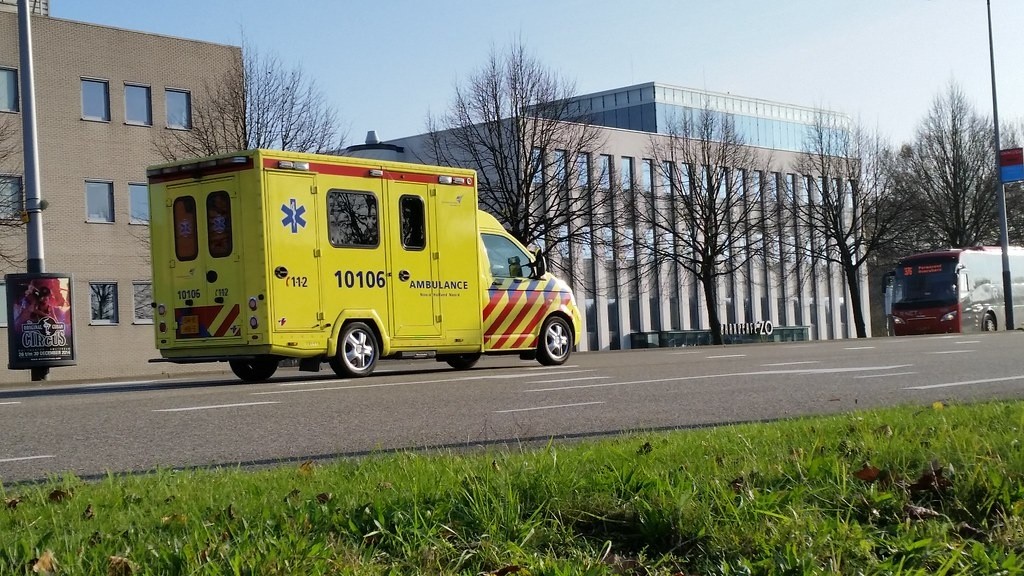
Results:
[880,245,1024,336]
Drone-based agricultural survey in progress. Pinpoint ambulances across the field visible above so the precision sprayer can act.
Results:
[145,149,582,383]
